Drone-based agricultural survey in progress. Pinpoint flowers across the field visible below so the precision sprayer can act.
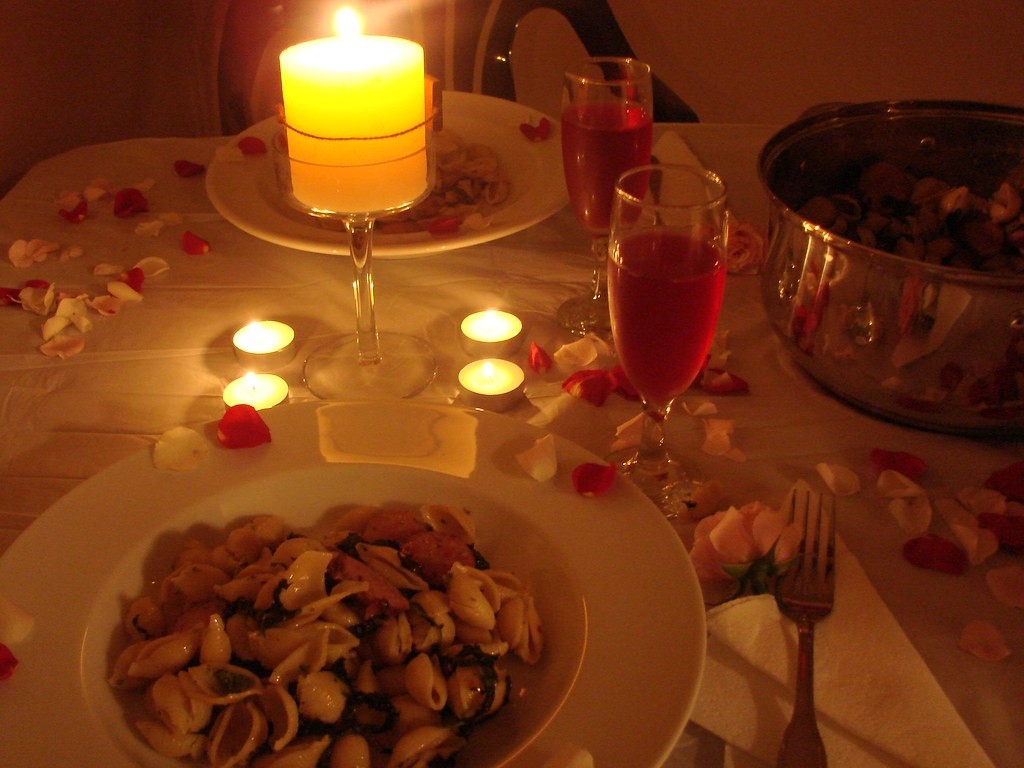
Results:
[704,205,768,275]
[687,496,801,597]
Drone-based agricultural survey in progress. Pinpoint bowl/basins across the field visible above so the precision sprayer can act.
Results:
[756,96,1024,436]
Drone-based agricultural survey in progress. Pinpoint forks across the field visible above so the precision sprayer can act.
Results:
[774,488,836,768]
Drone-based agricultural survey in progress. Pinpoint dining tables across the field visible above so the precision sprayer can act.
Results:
[1,125,1023,768]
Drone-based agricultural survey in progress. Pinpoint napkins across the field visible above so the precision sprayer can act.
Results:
[692,475,996,767]
[636,128,738,230]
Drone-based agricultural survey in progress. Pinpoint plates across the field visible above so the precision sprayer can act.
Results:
[0,401,707,768]
[204,89,583,260]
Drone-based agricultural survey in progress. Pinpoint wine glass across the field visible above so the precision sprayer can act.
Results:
[603,163,729,515]
[554,57,654,336]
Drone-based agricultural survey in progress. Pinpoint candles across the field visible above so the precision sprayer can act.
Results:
[278,3,430,211]
[458,356,524,413]
[223,372,289,413]
[460,308,522,360]
[233,319,297,370]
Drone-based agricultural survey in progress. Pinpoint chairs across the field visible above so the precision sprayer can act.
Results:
[216,1,700,124]
